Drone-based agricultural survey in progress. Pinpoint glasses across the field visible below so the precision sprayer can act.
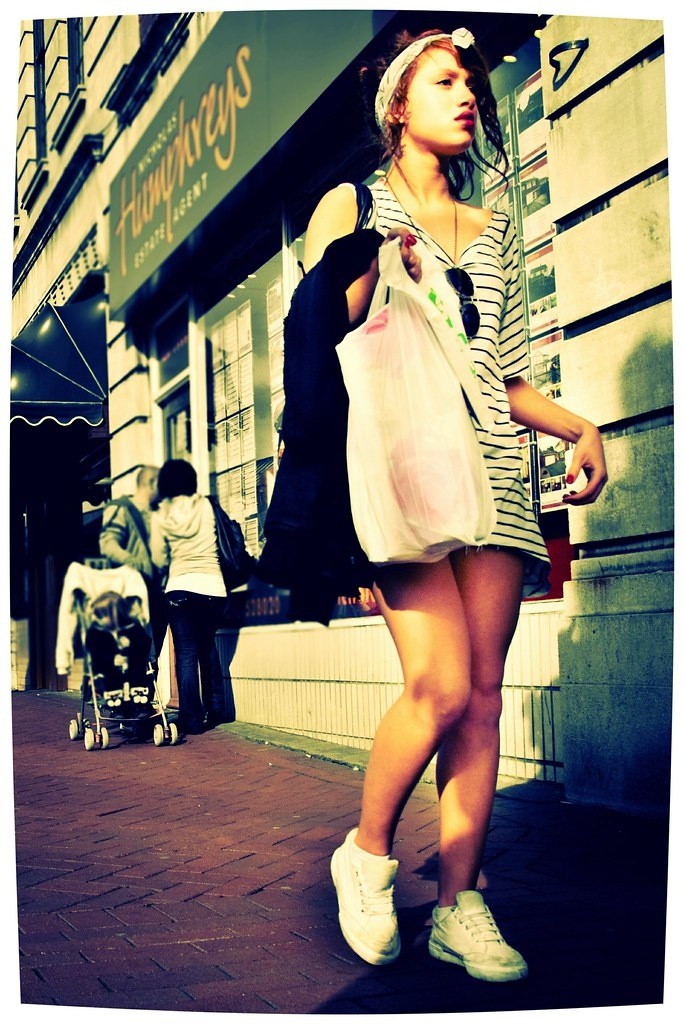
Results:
[446,265,480,341]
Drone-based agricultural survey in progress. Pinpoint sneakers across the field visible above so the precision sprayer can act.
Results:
[331,828,401,965]
[428,890,528,983]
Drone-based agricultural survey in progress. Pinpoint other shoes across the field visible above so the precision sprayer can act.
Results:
[168,719,202,734]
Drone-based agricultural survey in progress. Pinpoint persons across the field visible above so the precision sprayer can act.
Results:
[100,463,169,658]
[150,458,228,734]
[302,27,607,980]
[86,590,149,719]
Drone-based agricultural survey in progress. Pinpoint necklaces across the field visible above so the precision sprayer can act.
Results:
[386,180,457,268]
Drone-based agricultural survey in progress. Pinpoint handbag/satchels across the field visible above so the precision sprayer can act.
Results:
[206,495,259,592]
[334,236,497,564]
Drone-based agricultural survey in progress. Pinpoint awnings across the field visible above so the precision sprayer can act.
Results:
[10,295,107,426]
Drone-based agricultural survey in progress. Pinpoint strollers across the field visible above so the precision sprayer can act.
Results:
[69,562,178,751]
